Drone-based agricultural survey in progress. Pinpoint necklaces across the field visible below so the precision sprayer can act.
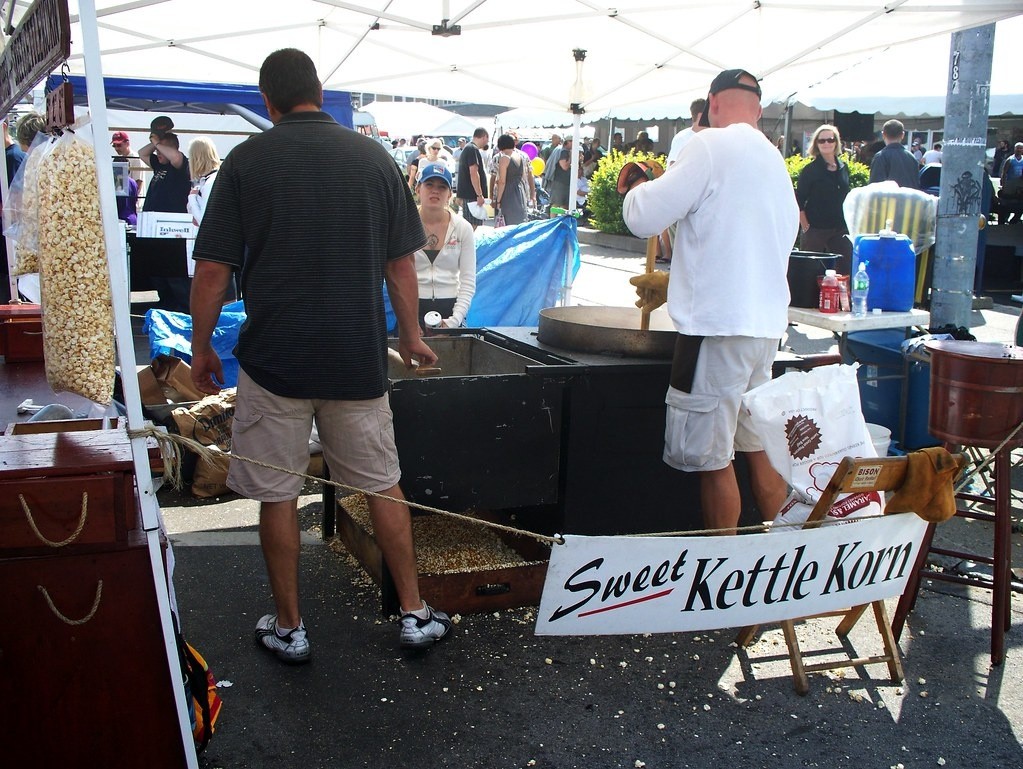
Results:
[420,211,446,250]
[825,160,837,168]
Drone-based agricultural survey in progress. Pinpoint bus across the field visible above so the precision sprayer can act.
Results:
[902,128,945,151]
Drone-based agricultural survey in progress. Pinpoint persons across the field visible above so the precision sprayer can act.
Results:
[191,49,453,660]
[870,120,920,193]
[138,117,237,316]
[655,98,710,271]
[622,71,800,536]
[387,128,656,235]
[796,125,852,260]
[394,165,476,337]
[0,113,55,305]
[772,134,1023,297]
[110,132,147,227]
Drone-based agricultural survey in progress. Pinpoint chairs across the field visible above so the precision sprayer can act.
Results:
[729,445,970,693]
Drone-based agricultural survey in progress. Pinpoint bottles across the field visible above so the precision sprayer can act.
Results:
[851,262,870,318]
[852,230,916,312]
[819,270,839,313]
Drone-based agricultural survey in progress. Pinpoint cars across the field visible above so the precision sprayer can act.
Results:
[388,146,458,191]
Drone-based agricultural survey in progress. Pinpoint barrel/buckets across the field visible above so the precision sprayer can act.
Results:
[787,251,843,308]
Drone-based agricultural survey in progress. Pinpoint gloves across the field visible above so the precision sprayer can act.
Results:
[630,270,670,314]
[616,160,665,194]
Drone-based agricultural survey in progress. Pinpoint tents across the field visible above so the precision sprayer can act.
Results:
[358,101,478,137]
[494,15,1023,160]
[44,74,353,133]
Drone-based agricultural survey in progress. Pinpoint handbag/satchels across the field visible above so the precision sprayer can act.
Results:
[583,162,597,176]
[467,198,491,221]
[494,209,505,228]
[137,354,212,405]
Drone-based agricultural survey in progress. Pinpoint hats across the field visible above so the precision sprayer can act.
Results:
[149,116,174,142]
[418,164,452,189]
[566,135,573,140]
[584,137,590,140]
[698,69,762,126]
[110,131,129,144]
[911,141,919,147]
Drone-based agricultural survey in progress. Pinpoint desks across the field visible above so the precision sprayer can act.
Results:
[781,295,991,448]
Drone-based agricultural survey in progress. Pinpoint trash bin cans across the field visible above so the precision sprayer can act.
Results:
[837,325,979,457]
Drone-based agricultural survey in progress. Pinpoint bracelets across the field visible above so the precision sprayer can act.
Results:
[496,198,501,205]
[154,141,161,151]
[476,193,483,197]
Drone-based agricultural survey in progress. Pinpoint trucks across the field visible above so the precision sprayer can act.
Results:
[33,87,393,199]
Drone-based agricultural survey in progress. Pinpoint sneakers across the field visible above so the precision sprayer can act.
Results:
[255,613,311,659]
[400,600,452,647]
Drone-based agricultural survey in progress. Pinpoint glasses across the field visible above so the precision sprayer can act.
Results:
[816,138,835,143]
[112,144,125,147]
[430,146,440,151]
